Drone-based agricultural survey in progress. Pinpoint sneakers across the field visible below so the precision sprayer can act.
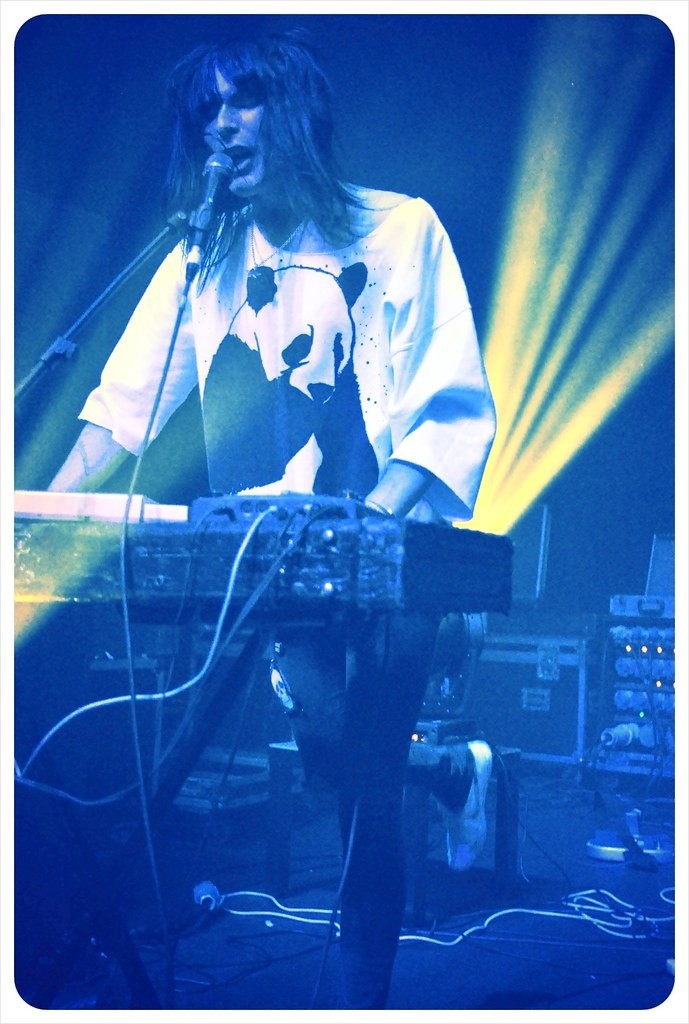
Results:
[436,739,492,867]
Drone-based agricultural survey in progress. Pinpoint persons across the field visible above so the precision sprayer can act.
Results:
[51,33,496,1010]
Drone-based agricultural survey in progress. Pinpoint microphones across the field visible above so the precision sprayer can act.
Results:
[184,153,232,280]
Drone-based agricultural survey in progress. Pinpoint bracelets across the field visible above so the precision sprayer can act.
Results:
[366,499,394,517]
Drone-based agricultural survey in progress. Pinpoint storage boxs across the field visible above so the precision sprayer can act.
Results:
[168,747,271,814]
[470,634,605,763]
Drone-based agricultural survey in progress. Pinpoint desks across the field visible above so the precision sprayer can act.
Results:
[267,742,521,929]
